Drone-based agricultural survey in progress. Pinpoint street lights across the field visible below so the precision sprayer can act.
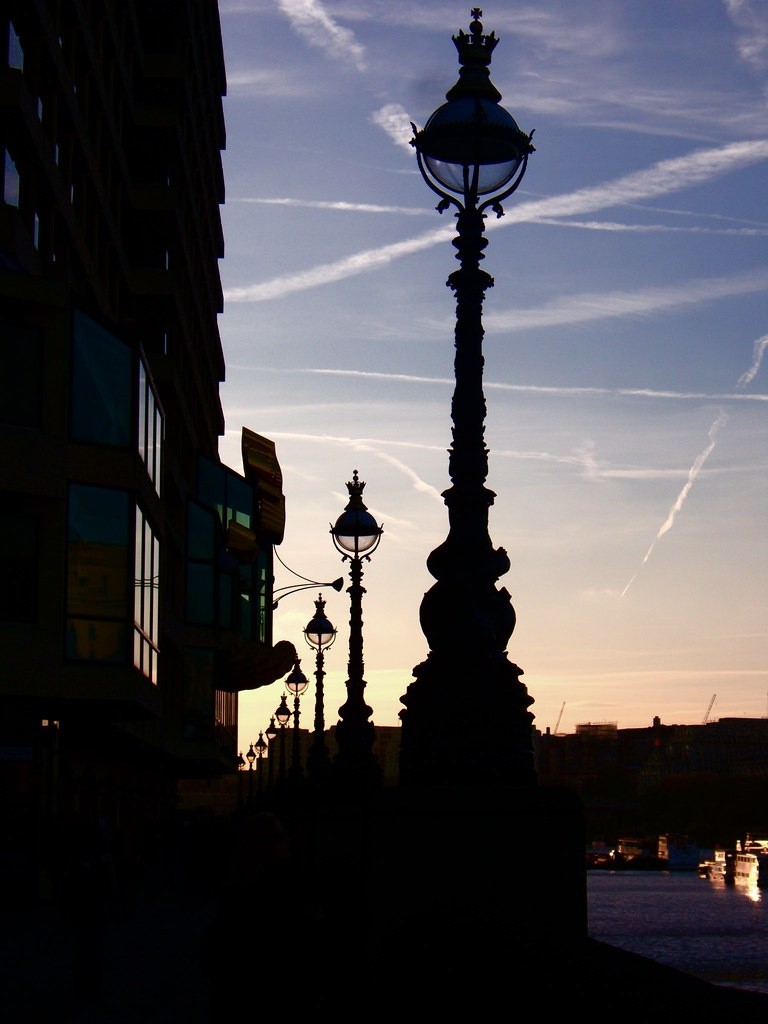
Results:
[397,0,539,804]
[327,467,387,798]
[265,718,278,780]
[275,695,292,785]
[246,745,256,775]
[254,733,268,786]
[285,657,308,780]
[304,593,338,776]
[238,752,245,781]
